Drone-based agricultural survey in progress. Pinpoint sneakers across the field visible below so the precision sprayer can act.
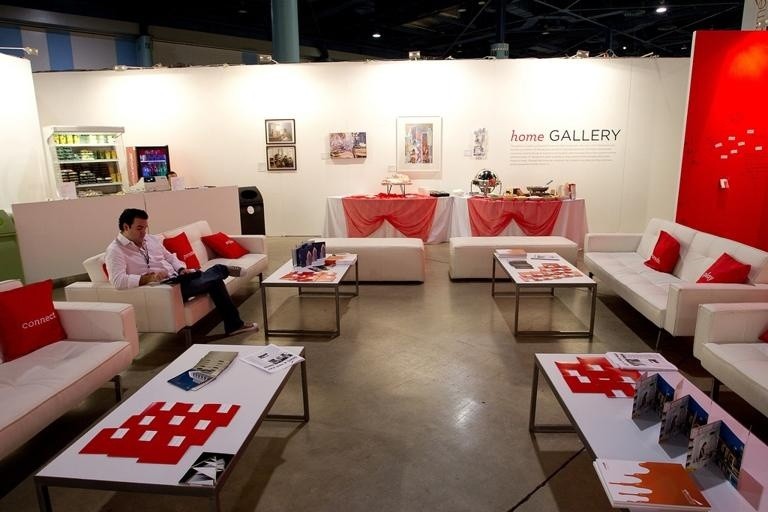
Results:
[225,321,257,336]
[227,266,246,278]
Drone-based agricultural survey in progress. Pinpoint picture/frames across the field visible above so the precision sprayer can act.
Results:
[265,118,296,145]
[266,145,297,171]
[395,115,443,174]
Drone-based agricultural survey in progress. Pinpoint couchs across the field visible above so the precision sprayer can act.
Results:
[692,302,768,418]
[583,216,768,353]
[0,280,141,464]
[64,220,269,349]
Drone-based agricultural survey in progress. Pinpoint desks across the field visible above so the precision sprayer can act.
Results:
[323,194,454,245]
[451,195,589,255]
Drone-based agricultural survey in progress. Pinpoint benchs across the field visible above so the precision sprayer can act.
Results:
[305,237,426,286]
[449,235,579,283]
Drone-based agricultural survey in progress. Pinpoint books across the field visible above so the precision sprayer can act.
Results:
[328,253,358,264]
[283,271,336,282]
[167,351,239,391]
[592,457,712,511]
[510,260,531,270]
[519,262,583,282]
[496,248,527,257]
[606,351,679,372]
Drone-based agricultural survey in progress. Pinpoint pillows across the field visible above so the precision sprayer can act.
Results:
[695,253,752,283]
[644,231,680,273]
[201,232,249,259]
[758,329,768,343]
[162,231,201,270]
[103,263,111,280]
[0,278,67,363]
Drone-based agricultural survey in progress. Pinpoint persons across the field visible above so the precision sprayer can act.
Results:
[105,208,258,335]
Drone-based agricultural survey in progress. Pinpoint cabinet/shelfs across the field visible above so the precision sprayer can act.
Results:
[42,124,131,199]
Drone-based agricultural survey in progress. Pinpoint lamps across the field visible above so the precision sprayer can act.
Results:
[0,46,39,56]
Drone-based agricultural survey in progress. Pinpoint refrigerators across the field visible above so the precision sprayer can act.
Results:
[126,146,171,186]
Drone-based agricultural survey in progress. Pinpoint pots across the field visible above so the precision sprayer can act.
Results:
[527,180,554,192]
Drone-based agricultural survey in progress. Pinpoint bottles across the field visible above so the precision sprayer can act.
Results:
[144,163,167,176]
